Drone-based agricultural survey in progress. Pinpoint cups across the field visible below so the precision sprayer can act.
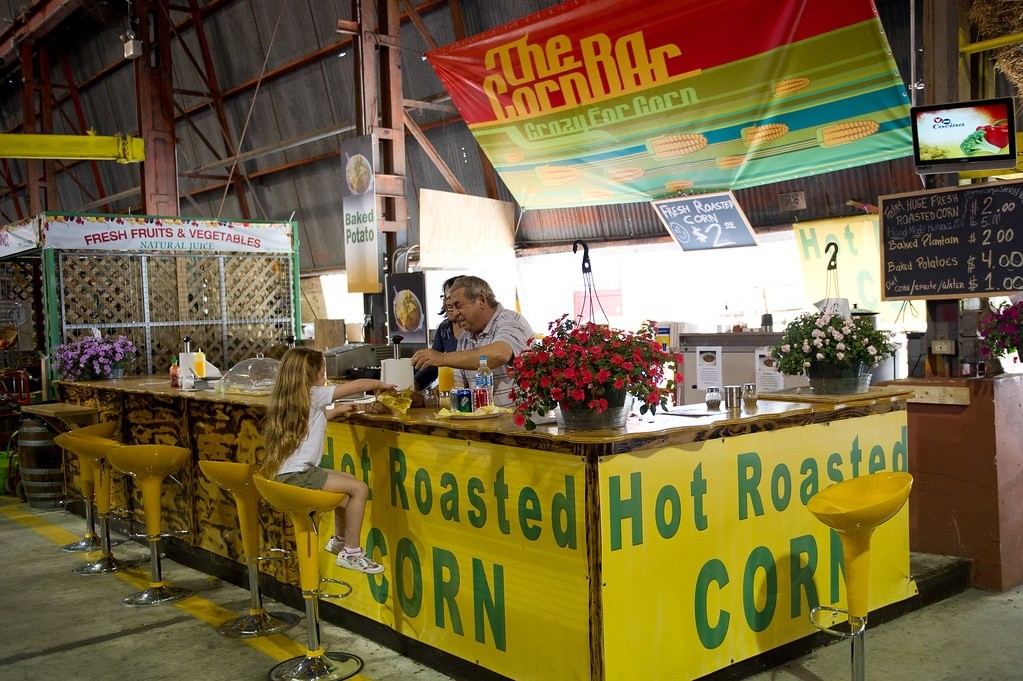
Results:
[717,324,723,333]
[178,374,194,389]
[724,385,741,408]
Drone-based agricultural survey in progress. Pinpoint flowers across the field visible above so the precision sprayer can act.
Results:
[976,301,1023,363]
[506,311,684,430]
[51,335,136,382]
[766,311,902,376]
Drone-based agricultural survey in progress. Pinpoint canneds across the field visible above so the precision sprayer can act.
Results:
[449,387,487,413]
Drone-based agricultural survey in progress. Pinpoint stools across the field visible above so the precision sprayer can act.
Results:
[807,471,914,681]
[199,460,300,639]
[251,474,364,681]
[57,420,123,553]
[105,443,196,607]
[54,432,142,575]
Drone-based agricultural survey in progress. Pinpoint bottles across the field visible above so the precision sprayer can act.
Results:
[475,356,495,406]
[169,356,180,387]
[449,386,459,413]
[742,383,758,406]
[439,391,451,411]
[438,365,455,391]
[193,348,206,377]
[705,387,721,410]
[424,389,438,409]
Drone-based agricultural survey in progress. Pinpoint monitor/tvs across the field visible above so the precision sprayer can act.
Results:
[911,95,1016,175]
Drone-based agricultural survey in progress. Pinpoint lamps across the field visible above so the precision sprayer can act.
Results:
[120,29,142,59]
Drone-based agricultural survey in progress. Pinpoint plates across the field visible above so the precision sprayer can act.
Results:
[450,412,500,420]
[761,358,776,370]
[701,352,716,364]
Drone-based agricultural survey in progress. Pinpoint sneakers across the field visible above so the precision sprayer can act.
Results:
[324,535,345,555]
[337,548,385,574]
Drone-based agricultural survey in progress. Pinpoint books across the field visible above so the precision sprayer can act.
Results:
[654,320,694,353]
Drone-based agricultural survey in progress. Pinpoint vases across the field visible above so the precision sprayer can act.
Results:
[806,362,871,394]
[559,378,632,429]
[1016,341,1023,362]
[106,368,124,379]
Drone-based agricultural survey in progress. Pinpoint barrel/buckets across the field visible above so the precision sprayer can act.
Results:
[17,418,64,508]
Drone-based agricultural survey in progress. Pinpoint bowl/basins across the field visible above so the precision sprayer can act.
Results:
[345,366,381,379]
[393,290,423,333]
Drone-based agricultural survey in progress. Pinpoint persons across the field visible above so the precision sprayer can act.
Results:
[257,347,398,574]
[412,276,553,414]
[415,275,467,391]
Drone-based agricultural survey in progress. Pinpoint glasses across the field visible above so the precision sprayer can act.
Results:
[440,295,447,300]
